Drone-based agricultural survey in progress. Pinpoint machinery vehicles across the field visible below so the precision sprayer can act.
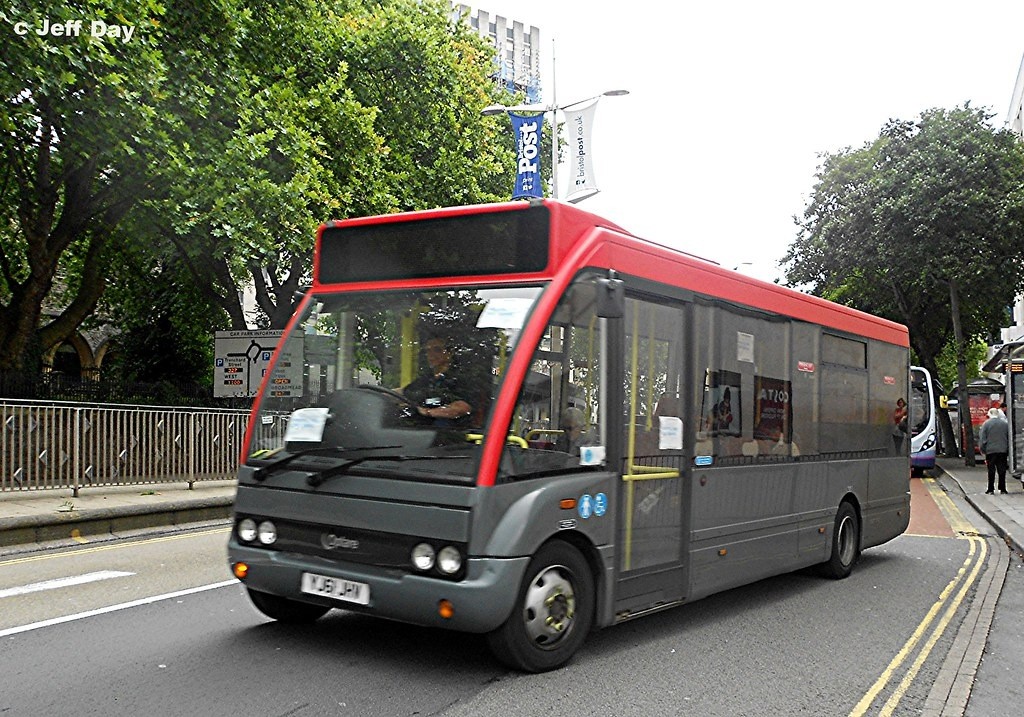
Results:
[911,363,941,479]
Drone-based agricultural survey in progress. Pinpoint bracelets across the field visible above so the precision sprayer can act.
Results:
[427,408,430,416]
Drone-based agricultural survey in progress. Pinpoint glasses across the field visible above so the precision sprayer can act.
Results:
[561,426,574,431]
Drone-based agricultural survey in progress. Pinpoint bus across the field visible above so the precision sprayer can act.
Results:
[224,197,919,679]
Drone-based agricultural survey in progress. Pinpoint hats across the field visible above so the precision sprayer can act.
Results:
[987,408,999,419]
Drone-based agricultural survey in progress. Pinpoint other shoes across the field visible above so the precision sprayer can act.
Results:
[985,490,994,495]
[1000,489,1008,494]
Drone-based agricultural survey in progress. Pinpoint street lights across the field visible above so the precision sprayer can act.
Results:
[477,37,636,208]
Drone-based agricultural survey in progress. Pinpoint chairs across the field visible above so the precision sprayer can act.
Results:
[524,382,899,457]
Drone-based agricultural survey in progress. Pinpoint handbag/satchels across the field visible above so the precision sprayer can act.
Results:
[899,418,908,434]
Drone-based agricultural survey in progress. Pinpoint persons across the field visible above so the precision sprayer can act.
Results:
[998,403,1007,421]
[557,407,584,457]
[718,387,732,430]
[980,407,1008,495]
[383,332,478,426]
[892,398,907,455]
